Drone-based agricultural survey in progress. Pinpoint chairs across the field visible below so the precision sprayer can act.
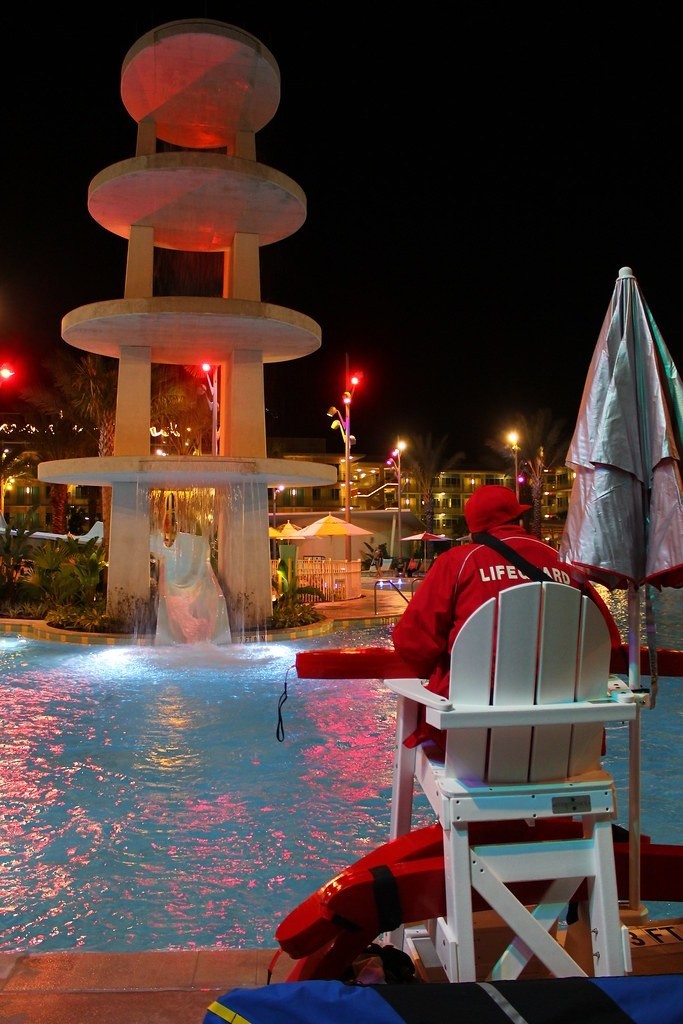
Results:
[383,581,651,985]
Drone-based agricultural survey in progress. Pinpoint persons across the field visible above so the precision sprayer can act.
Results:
[392,485,622,756]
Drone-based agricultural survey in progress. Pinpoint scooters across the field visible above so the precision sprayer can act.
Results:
[361,542,389,571]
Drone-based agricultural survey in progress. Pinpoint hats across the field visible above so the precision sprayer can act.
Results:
[463,485,532,533]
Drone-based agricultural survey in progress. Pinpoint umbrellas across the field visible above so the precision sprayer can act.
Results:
[400,530,453,572]
[276,519,322,545]
[269,527,287,539]
[290,511,375,543]
[555,267,683,909]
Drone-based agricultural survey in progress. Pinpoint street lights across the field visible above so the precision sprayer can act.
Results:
[503,428,521,527]
[273,486,285,561]
[385,433,407,577]
[325,352,360,558]
[203,362,220,457]
